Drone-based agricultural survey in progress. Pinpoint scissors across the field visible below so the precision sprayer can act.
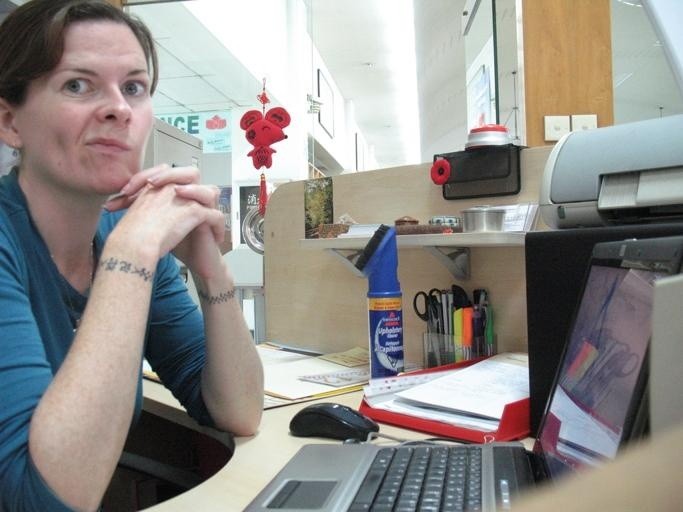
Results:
[578,341,641,402]
[413,287,442,367]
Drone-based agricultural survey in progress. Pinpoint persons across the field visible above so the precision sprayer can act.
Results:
[0,1,262,512]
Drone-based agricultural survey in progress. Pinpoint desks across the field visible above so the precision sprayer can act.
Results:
[116,117,681,512]
[217,243,265,345]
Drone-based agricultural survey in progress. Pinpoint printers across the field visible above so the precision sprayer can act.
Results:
[538,112,683,229]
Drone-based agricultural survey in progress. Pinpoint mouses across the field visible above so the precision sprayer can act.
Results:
[290,402,379,441]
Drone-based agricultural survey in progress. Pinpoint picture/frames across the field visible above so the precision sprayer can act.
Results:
[317,69,334,138]
[356,130,367,172]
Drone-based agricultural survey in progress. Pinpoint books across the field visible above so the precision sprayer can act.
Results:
[543,383,624,461]
[393,351,529,422]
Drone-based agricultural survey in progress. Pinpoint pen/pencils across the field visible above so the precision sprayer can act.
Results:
[560,329,623,408]
[427,285,494,368]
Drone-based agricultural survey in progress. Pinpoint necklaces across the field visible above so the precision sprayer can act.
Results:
[48,239,95,334]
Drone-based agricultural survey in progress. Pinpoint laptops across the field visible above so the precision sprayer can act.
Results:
[242,235,683,512]
